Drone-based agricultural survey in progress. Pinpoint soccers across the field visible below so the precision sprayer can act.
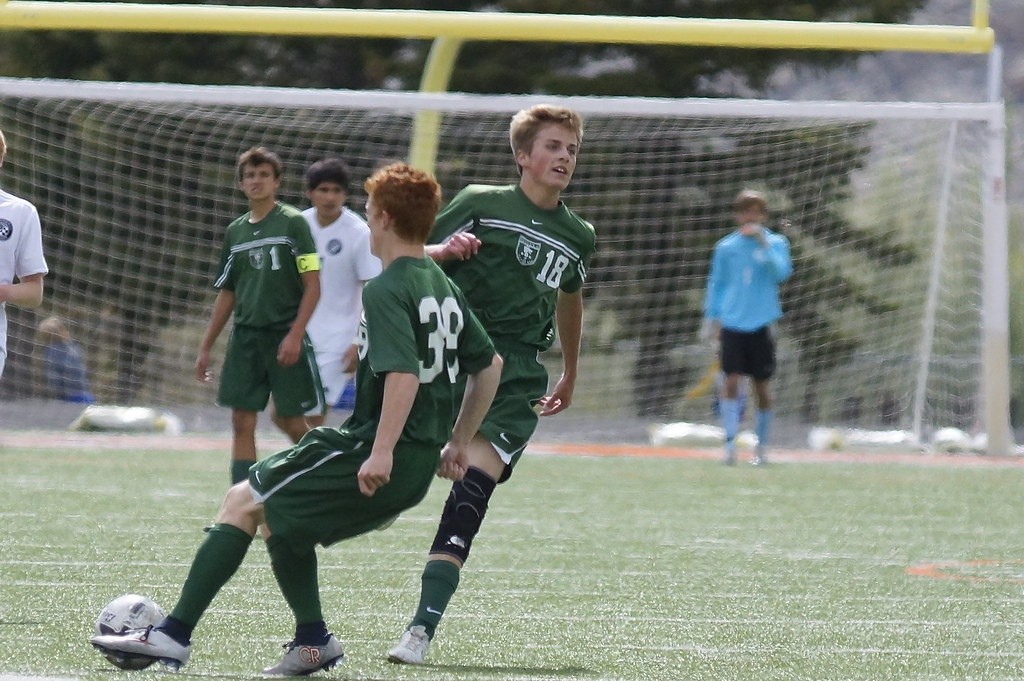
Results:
[93,594,170,670]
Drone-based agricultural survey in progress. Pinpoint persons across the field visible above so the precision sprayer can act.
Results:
[88,104,598,675]
[0,128,96,431]
[697,191,793,467]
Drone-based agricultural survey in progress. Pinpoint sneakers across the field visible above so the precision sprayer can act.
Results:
[718,449,738,468]
[748,450,771,467]
[388,624,433,664]
[90,625,192,667]
[264,635,343,679]
